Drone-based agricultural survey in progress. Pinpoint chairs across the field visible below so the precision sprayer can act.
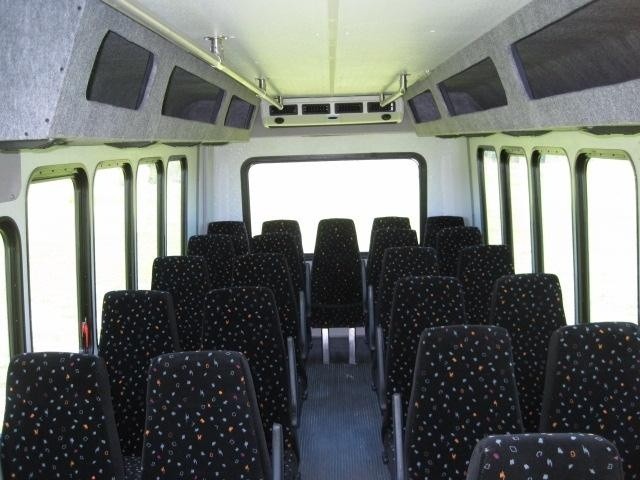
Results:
[0,215,640,480]
